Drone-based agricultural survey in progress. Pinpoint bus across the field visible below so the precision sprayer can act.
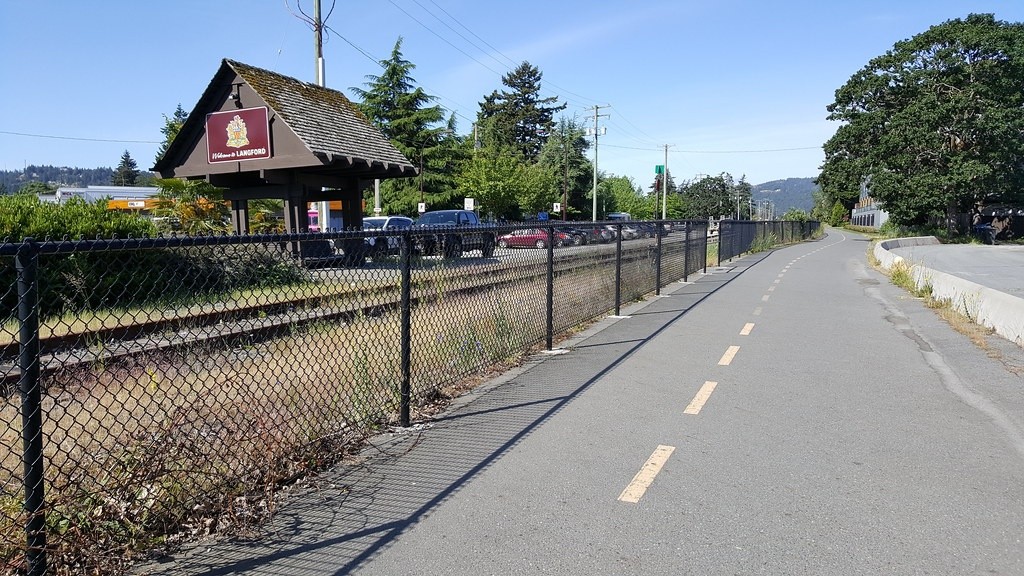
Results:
[608,212,632,223]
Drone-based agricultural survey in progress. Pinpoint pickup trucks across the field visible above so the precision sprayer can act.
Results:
[407,210,499,260]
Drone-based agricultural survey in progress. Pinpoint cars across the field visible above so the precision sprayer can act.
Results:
[356,214,414,261]
[496,221,693,249]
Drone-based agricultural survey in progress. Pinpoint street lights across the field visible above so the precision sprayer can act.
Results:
[420,128,454,207]
